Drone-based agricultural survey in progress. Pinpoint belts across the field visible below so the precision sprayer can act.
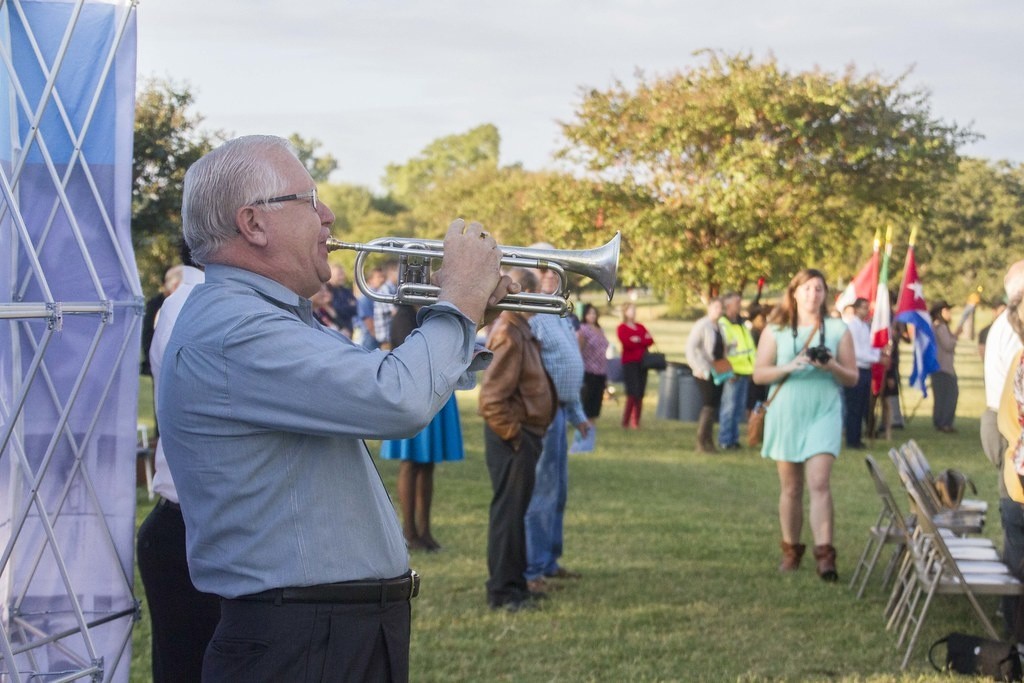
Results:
[239,569,423,604]
[158,495,184,512]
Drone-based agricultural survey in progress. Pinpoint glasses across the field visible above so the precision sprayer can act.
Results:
[243,190,317,223]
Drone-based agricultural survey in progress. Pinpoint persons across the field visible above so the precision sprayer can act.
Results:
[615,300,654,429]
[747,267,861,580]
[832,292,903,449]
[159,133,523,683]
[134,230,221,682]
[687,292,775,453]
[978,259,1024,653]
[474,238,592,609]
[577,303,608,423]
[928,302,966,433]
[313,259,467,554]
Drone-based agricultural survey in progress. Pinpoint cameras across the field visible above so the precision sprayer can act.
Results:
[807,347,830,363]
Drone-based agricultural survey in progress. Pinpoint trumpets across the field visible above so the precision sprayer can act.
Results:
[325,229,622,319]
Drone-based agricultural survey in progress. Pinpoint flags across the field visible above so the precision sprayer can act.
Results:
[869,245,895,396]
[896,250,943,398]
[833,253,876,321]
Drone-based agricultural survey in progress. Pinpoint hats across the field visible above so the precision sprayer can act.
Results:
[929,299,956,317]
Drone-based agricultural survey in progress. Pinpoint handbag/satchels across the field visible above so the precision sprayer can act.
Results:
[928,631,1024,683]
[709,360,736,385]
[745,401,770,445]
[640,341,666,370]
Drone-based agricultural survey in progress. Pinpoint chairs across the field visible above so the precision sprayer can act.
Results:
[849,438,1024,671]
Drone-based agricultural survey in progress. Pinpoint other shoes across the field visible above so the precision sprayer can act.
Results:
[718,440,741,453]
[401,536,439,555]
[500,590,553,613]
[936,425,959,435]
[550,564,585,579]
[525,575,564,594]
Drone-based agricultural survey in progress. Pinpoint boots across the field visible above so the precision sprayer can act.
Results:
[696,405,721,456]
[780,541,807,573]
[621,394,637,431]
[809,542,841,580]
[629,398,643,430]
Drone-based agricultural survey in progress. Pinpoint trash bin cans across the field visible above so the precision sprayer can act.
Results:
[656,361,707,421]
